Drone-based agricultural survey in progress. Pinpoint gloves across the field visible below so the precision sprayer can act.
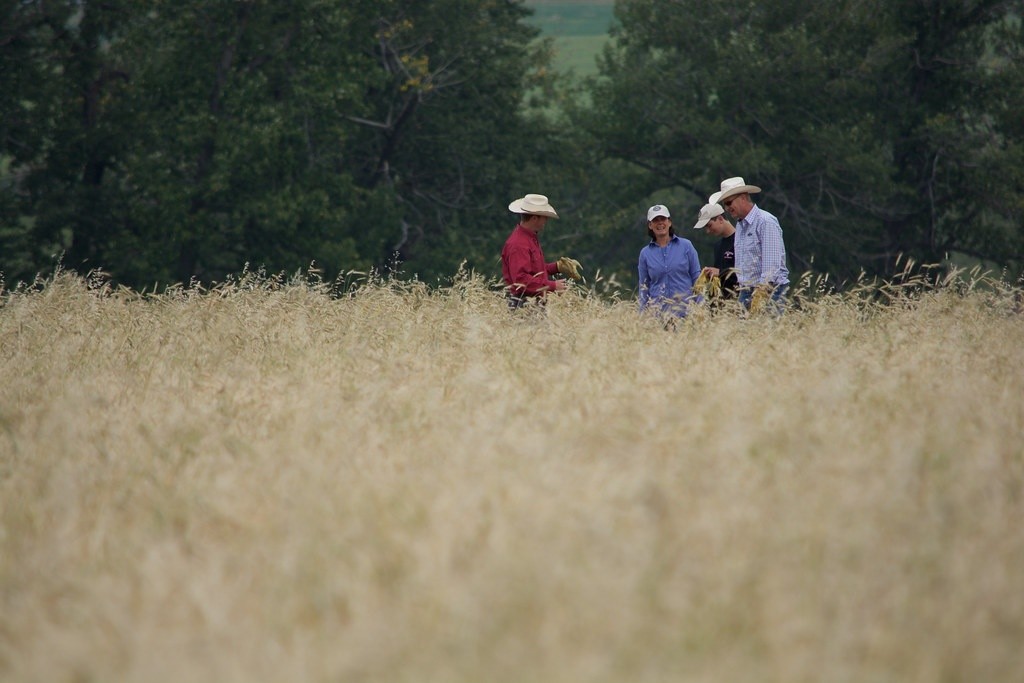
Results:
[706,276,721,298]
[750,288,768,310]
[693,269,707,295]
[556,257,583,281]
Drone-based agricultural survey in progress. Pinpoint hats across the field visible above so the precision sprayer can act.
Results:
[693,204,725,228]
[709,177,761,207]
[647,205,670,221]
[508,194,559,219]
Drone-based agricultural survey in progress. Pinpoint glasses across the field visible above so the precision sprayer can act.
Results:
[722,194,741,209]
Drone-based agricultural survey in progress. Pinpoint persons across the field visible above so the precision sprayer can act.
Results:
[502,194,583,309]
[694,204,736,306]
[635,205,705,317]
[708,177,790,318]
